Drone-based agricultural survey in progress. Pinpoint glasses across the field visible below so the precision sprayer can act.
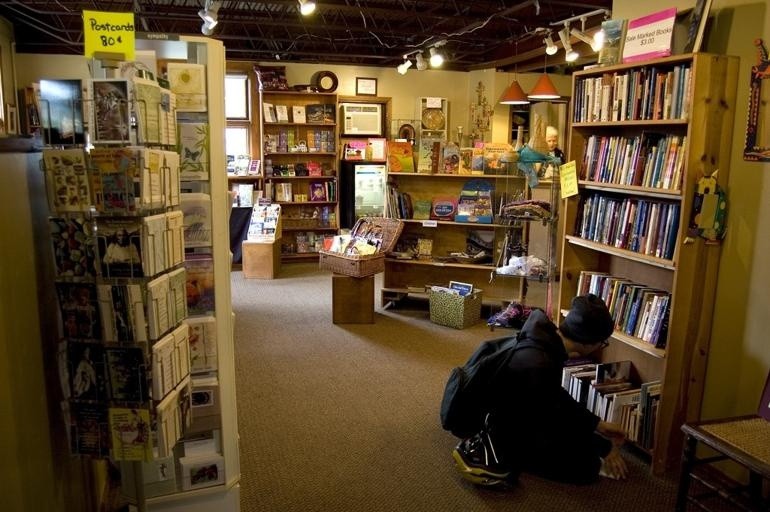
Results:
[600,338,610,350]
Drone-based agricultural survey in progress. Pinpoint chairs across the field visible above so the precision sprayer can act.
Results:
[677,371,770,512]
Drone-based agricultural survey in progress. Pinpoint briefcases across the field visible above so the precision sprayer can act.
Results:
[320,216,405,277]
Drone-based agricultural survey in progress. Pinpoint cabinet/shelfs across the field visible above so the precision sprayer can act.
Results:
[259,90,340,264]
[416,97,448,142]
[24,86,42,135]
[87,35,241,512]
[242,240,280,279]
[555,51,742,477]
[332,270,376,324]
[380,172,529,310]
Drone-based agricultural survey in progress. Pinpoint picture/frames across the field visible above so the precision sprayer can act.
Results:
[355,76,378,97]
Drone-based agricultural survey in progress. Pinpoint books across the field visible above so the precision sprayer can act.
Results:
[561,359,660,450]
[89,57,225,501]
[574,194,681,259]
[573,64,691,123]
[42,147,108,464]
[383,137,528,272]
[682,0,712,54]
[431,284,460,297]
[596,19,630,65]
[579,130,687,190]
[578,271,672,350]
[449,280,473,297]
[227,102,341,255]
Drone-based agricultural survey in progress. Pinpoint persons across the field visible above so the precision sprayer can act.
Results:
[545,126,567,165]
[437,294,632,495]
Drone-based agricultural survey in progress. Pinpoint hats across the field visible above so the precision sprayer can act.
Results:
[567,293,613,344]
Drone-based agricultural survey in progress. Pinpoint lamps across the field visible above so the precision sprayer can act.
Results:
[499,24,530,107]
[533,9,612,64]
[297,0,318,16]
[527,25,563,101]
[396,38,450,76]
[197,1,224,37]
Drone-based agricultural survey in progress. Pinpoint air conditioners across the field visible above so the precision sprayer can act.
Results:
[341,103,384,135]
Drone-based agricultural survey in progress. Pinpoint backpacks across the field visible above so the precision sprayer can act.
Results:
[441,333,542,438]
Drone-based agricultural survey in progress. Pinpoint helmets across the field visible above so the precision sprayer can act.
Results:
[454,432,511,490]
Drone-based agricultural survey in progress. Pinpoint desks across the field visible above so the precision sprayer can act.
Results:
[228,174,261,191]
